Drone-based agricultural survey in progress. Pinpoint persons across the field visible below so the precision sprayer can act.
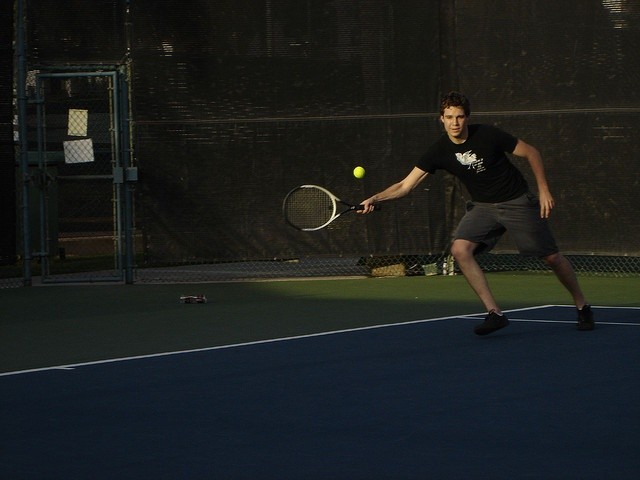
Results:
[353,91,595,336]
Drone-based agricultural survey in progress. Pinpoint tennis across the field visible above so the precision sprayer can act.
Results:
[353,167,365,178]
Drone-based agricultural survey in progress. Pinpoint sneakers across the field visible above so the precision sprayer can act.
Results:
[575,304,596,330]
[474,309,510,335]
